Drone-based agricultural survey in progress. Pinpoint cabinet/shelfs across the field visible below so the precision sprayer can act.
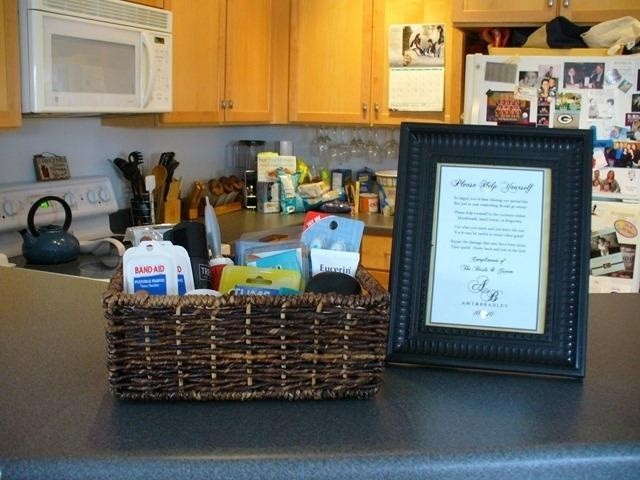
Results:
[1,0,638,130]
[360,229,393,293]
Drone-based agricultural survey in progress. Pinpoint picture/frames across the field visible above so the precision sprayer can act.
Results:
[385,120,594,380]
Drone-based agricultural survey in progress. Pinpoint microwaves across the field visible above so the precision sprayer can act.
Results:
[16,0,171,113]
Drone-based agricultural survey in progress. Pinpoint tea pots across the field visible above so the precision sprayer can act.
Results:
[16,196,80,265]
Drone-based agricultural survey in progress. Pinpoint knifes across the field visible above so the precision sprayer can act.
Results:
[159,151,180,180]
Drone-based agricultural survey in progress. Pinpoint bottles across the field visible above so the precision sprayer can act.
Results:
[204,174,242,206]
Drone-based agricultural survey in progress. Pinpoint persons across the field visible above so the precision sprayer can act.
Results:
[517,61,606,127]
[410,26,445,59]
[593,140,640,195]
[597,237,612,257]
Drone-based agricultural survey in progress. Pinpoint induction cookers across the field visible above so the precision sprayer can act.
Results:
[21,235,129,279]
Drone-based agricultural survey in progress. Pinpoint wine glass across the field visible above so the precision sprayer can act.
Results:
[308,121,399,167]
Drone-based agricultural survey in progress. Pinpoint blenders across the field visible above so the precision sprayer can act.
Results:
[233,136,263,209]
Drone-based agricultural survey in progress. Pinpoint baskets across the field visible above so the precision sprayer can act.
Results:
[103,253,392,401]
